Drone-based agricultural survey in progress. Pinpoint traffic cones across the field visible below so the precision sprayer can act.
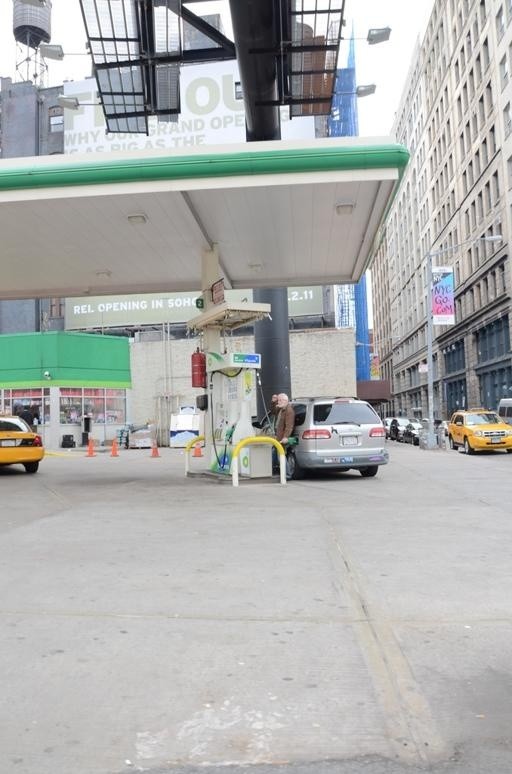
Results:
[84,435,97,458]
[150,440,162,459]
[110,439,119,458]
[192,437,204,458]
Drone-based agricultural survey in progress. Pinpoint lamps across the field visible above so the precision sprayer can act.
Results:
[282,82,377,98]
[56,93,144,110]
[281,24,391,46]
[39,44,146,61]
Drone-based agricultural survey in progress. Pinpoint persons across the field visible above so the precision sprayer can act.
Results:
[34,413,41,424]
[21,406,33,429]
[271,393,295,465]
[391,419,399,426]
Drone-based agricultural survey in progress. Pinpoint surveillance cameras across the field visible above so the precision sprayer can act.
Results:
[44,371,49,377]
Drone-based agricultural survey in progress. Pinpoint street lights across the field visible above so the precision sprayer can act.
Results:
[357,334,404,380]
[425,235,503,452]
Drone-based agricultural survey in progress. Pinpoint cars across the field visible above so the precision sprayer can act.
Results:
[446,407,512,453]
[383,415,452,444]
[60,406,125,424]
[496,398,512,424]
[0,415,46,477]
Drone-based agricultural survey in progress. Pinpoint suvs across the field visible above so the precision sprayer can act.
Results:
[253,393,389,479]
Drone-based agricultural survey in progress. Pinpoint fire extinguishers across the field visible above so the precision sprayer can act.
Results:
[191,347,207,388]
